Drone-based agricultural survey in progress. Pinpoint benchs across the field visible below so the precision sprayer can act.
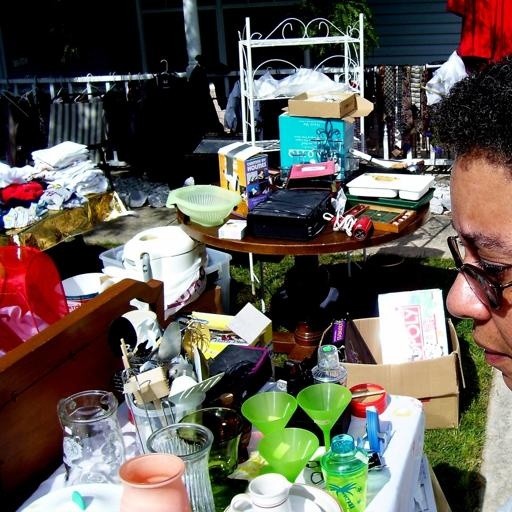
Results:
[0,278,165,490]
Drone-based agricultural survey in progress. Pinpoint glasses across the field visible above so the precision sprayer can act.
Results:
[447,235,512,310]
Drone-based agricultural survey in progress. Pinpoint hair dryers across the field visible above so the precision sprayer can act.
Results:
[322,210,371,241]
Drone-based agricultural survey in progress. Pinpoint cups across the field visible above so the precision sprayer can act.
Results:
[58,390,215,512]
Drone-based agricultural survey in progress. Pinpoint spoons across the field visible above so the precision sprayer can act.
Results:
[157,322,181,363]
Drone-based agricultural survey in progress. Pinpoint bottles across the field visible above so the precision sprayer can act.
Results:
[320,434,367,511]
[312,345,347,387]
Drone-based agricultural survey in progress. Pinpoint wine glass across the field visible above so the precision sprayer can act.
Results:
[175,384,354,512]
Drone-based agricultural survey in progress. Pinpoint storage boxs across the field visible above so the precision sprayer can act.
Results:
[338,316,467,430]
[278,91,375,179]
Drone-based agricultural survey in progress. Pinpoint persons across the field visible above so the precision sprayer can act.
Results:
[428,55,512,512]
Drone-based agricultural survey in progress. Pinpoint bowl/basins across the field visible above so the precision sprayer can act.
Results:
[349,384,387,417]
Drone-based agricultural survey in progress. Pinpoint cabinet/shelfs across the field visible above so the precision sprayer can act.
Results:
[237,12,365,156]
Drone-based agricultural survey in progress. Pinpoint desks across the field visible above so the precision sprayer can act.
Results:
[15,386,424,511]
[179,178,430,310]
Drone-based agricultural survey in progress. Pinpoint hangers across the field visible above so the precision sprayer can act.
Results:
[1,58,170,122]
